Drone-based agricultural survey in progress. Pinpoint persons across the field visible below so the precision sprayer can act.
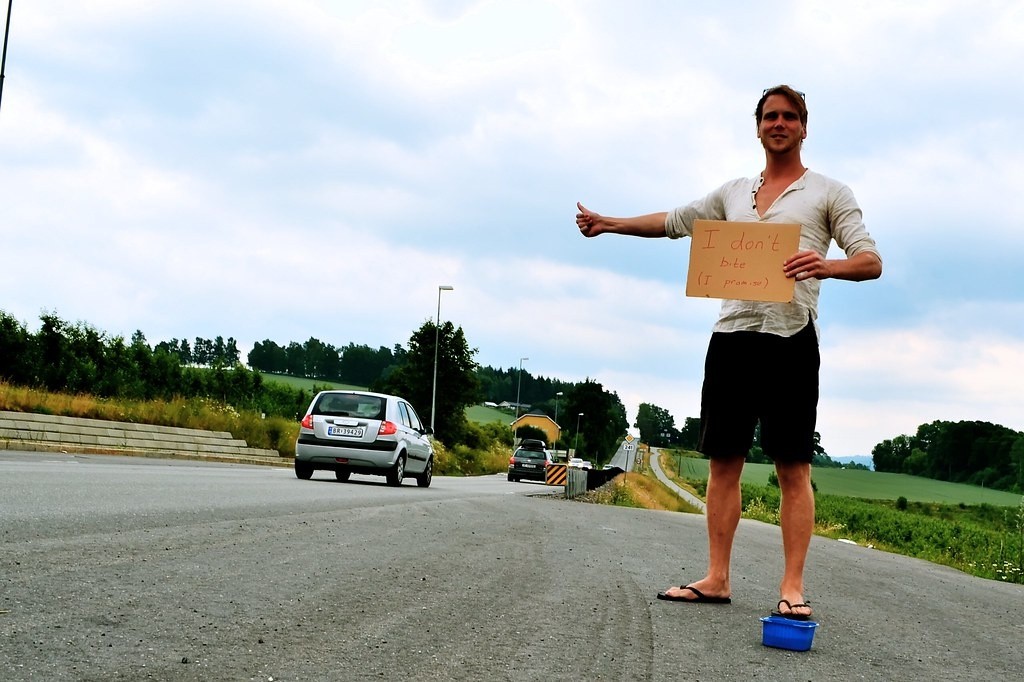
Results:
[577,85,882,619]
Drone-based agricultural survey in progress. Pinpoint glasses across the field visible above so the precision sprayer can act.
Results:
[763,89,805,100]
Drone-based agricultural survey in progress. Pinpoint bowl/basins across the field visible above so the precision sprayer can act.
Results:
[758,615,819,652]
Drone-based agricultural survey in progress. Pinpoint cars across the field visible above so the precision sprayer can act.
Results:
[294,390,434,488]
[568,457,593,470]
[603,464,614,470]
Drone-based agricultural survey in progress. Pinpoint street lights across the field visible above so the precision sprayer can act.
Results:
[555,392,564,423]
[515,357,529,450]
[430,286,454,432]
[575,413,585,451]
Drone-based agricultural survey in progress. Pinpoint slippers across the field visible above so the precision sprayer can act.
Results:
[657,584,731,604]
[771,599,811,620]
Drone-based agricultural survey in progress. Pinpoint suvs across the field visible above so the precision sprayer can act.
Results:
[508,438,560,484]
